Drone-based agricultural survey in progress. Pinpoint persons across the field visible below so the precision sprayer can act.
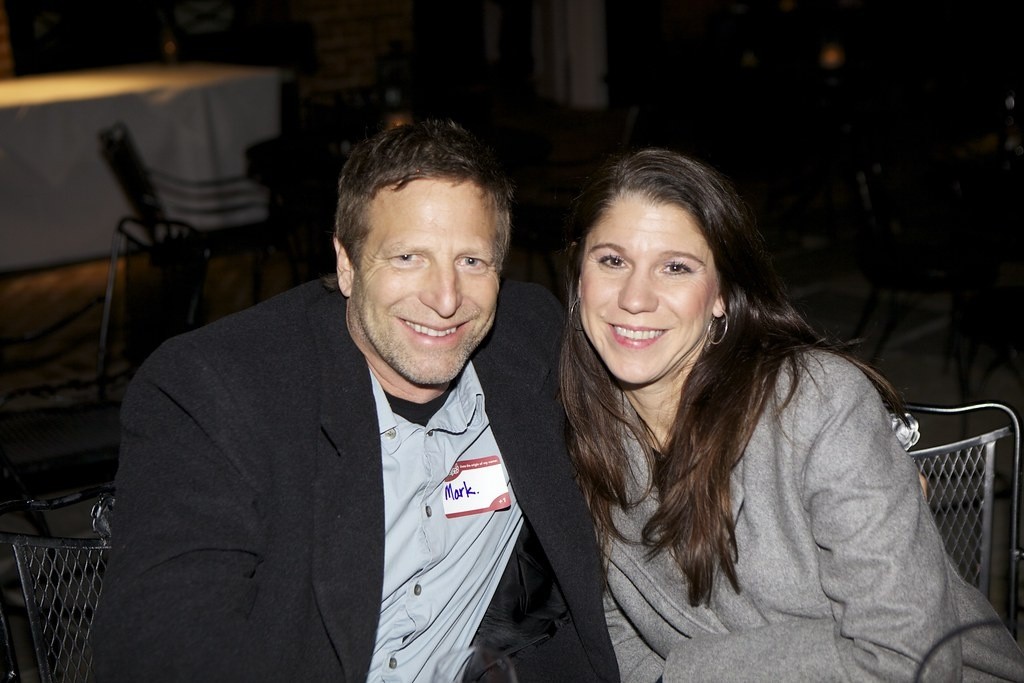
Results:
[557,146,1024,683]
[87,117,926,683]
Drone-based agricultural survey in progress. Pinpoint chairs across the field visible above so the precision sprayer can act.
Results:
[0,480,115,683]
[890,400,1024,639]
[95,119,280,402]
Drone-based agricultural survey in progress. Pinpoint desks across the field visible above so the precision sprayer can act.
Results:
[0,62,298,269]
[247,121,553,288]
